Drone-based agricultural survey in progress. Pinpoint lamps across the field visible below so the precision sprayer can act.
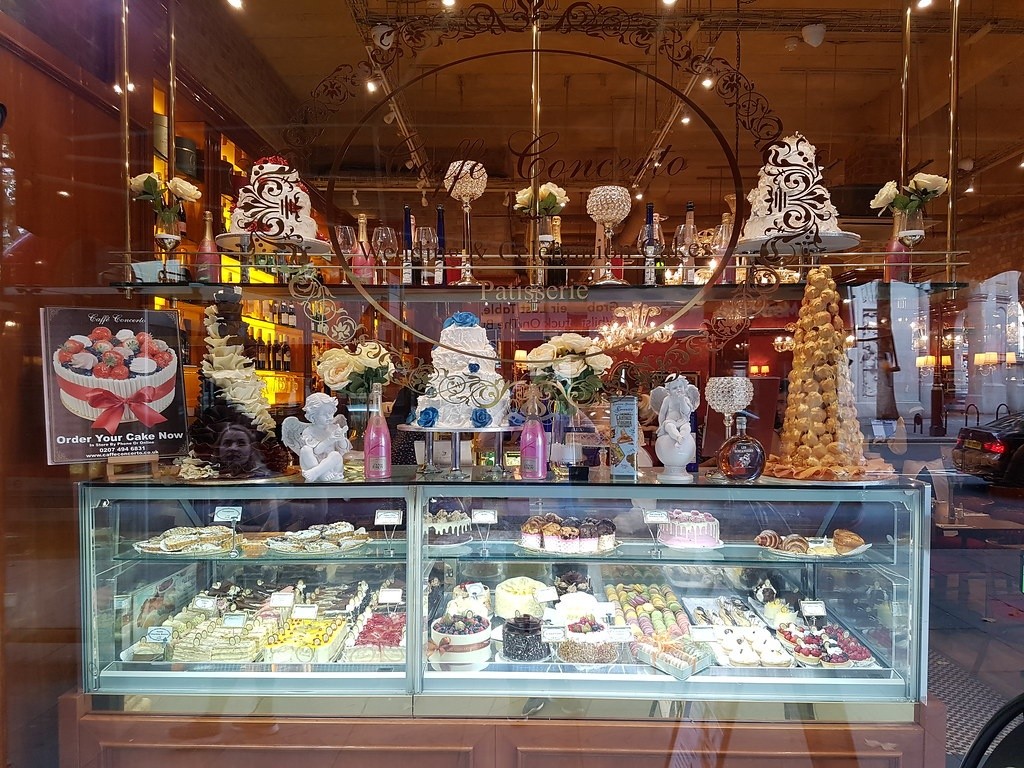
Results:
[383,112,394,124]
[421,192,428,206]
[368,81,382,92]
[415,180,425,190]
[750,365,769,377]
[1005,352,1016,369]
[634,190,643,199]
[942,356,951,371]
[915,355,935,376]
[974,351,998,375]
[592,302,677,357]
[352,190,359,206]
[405,160,415,169]
[663,260,731,285]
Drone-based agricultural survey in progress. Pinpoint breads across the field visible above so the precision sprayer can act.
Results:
[754,529,864,555]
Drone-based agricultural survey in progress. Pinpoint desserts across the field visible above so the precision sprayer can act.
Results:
[521,513,617,554]
[137,521,369,550]
[696,582,871,668]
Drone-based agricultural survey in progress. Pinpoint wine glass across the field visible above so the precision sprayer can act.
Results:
[332,226,357,285]
[637,224,665,286]
[712,225,734,284]
[898,208,925,283]
[672,225,697,286]
[538,216,552,285]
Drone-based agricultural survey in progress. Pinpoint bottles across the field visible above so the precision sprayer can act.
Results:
[178,201,187,236]
[241,298,327,371]
[156,172,167,211]
[414,227,439,285]
[719,417,766,482]
[404,333,409,354]
[255,252,323,283]
[351,213,374,285]
[176,309,190,364]
[521,384,547,479]
[547,216,564,285]
[370,227,397,285]
[364,383,391,479]
[195,211,220,282]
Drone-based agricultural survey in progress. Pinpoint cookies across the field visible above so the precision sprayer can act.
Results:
[605,583,706,670]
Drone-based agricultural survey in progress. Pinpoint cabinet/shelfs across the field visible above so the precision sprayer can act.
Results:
[73,478,933,701]
[151,149,279,278]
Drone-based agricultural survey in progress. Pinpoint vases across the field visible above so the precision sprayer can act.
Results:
[154,215,182,279]
[343,396,371,480]
[524,213,552,285]
[551,400,582,479]
[899,209,925,284]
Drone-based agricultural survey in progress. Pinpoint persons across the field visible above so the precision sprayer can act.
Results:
[198,426,280,589]
[650,373,700,448]
[888,387,959,537]
[282,392,347,482]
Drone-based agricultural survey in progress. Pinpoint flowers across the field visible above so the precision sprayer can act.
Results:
[126,173,202,217]
[320,340,392,393]
[525,332,612,396]
[869,170,947,214]
[514,181,569,217]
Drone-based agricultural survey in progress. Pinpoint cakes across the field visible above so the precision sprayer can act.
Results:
[429,571,618,664]
[233,564,406,589]
[742,131,842,239]
[230,156,319,237]
[426,509,471,545]
[53,327,178,422]
[413,311,511,428]
[657,509,720,548]
[130,579,407,663]
